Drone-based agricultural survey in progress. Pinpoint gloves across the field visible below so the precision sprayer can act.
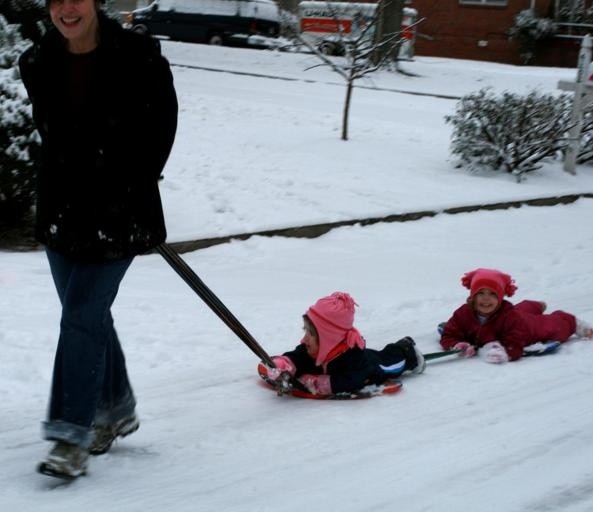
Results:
[453,341,509,364]
[297,374,333,396]
[258,355,296,387]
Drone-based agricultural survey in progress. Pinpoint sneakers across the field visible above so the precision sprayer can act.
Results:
[36,411,140,480]
[404,335,425,375]
[574,319,592,338]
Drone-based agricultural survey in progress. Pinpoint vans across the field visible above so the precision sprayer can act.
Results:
[123,0,281,49]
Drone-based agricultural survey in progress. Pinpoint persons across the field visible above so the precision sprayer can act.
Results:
[17,0,178,480]
[437,267,593,364]
[258,290,424,395]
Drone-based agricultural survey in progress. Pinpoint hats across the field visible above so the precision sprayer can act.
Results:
[306,291,354,366]
[461,268,516,315]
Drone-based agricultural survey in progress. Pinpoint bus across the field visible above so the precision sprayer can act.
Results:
[291,1,416,60]
